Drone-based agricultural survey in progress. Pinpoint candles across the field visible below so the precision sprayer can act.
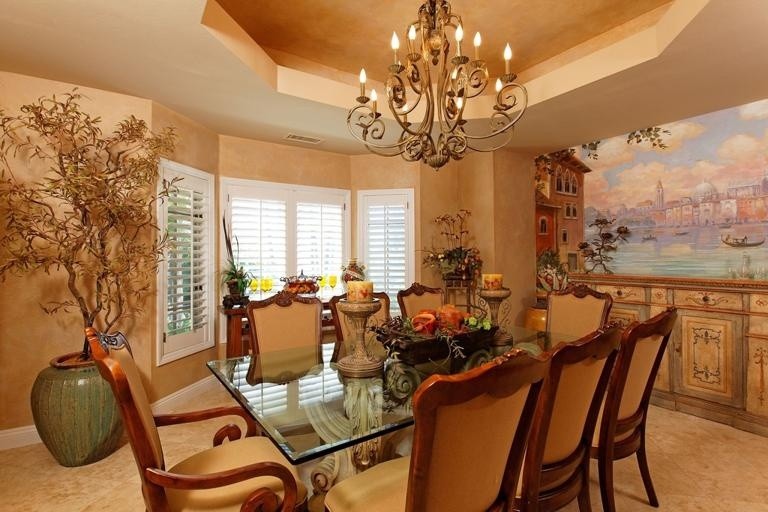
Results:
[482,273,503,290]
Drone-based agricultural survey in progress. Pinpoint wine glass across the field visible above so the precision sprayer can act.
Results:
[249,279,258,301]
[319,276,325,300]
[329,275,337,297]
[260,279,272,300]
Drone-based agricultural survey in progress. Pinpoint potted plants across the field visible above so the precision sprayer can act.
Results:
[213,257,258,295]
[0,87,185,469]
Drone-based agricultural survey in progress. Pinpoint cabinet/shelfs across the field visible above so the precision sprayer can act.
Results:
[671,287,745,412]
[742,287,768,420]
[443,280,476,314]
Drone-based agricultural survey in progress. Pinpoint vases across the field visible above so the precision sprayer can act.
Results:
[222,294,249,307]
[368,322,499,368]
[445,267,469,287]
[340,257,366,286]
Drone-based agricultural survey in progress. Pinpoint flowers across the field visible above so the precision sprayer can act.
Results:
[370,306,496,360]
[414,208,483,281]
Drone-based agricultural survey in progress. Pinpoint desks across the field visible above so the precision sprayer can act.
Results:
[216,301,334,356]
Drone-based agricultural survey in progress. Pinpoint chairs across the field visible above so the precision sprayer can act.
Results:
[511,319,622,512]
[592,303,675,512]
[331,291,393,339]
[543,281,618,335]
[398,280,443,320]
[85,325,311,511]
[322,347,540,512]
[246,291,321,351]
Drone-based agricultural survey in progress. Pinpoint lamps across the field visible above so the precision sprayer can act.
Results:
[342,2,529,170]
